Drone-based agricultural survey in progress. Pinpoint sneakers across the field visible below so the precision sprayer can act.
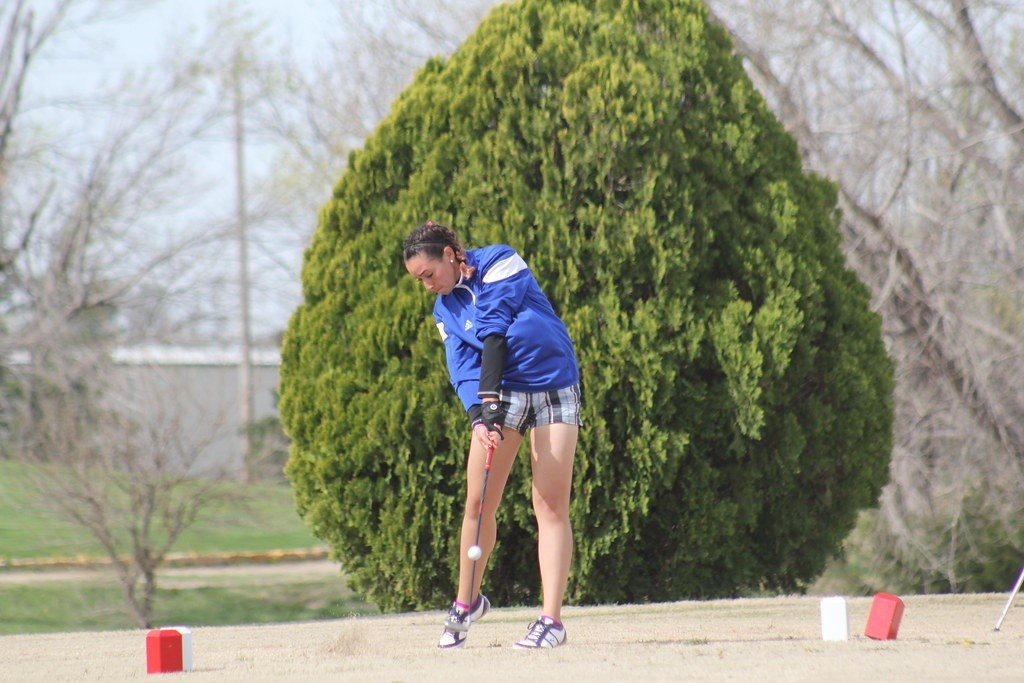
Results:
[513,615,568,650]
[434,592,491,650]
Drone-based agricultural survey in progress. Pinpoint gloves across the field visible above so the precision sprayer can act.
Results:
[481,401,505,440]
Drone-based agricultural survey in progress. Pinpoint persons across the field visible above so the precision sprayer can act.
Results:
[399,224,582,651]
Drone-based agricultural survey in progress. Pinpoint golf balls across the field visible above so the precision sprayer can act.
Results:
[467,545,482,560]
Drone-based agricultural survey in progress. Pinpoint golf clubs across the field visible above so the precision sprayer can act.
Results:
[442,389,503,632]
